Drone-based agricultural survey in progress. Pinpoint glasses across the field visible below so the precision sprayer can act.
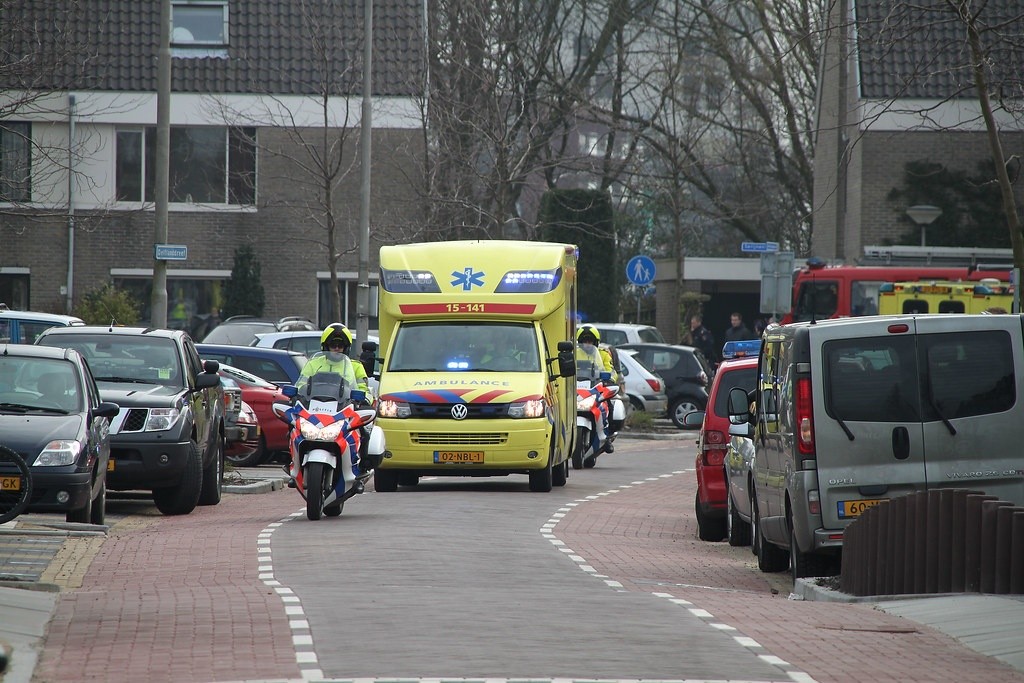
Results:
[582,337,595,343]
[329,342,344,350]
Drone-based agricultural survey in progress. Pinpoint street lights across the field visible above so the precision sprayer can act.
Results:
[906,205,942,246]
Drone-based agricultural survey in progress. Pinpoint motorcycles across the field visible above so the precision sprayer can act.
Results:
[575,343,621,471]
[273,352,387,514]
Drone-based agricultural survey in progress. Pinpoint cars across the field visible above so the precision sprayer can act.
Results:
[596,341,671,423]
[200,359,295,463]
[248,332,382,398]
[576,323,672,371]
[615,343,716,430]
[0,342,123,525]
[126,343,307,393]
[683,339,763,543]
[718,388,762,556]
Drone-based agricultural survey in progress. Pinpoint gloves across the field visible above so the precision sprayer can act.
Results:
[355,397,369,406]
[291,394,306,405]
[604,379,615,386]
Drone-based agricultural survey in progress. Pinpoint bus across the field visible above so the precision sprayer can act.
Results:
[781,259,1024,326]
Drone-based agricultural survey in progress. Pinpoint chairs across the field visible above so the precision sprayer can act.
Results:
[34,372,75,410]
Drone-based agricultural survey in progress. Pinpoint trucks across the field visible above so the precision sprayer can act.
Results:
[875,276,1018,315]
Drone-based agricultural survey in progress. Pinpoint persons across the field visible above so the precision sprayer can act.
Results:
[288,323,373,493]
[681,315,714,356]
[577,324,618,454]
[481,329,521,364]
[726,312,752,341]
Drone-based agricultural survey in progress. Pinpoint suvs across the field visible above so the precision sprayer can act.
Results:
[16,324,228,512]
[0,309,134,359]
[201,314,318,345]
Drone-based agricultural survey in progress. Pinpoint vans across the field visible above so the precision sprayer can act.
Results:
[724,312,1024,587]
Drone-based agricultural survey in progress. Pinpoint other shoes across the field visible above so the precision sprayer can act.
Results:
[287,477,297,488]
[603,438,614,453]
[356,480,364,493]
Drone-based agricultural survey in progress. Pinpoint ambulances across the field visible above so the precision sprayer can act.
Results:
[361,239,581,495]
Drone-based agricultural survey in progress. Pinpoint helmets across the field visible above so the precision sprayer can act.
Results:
[320,322,353,353]
[576,323,600,347]
